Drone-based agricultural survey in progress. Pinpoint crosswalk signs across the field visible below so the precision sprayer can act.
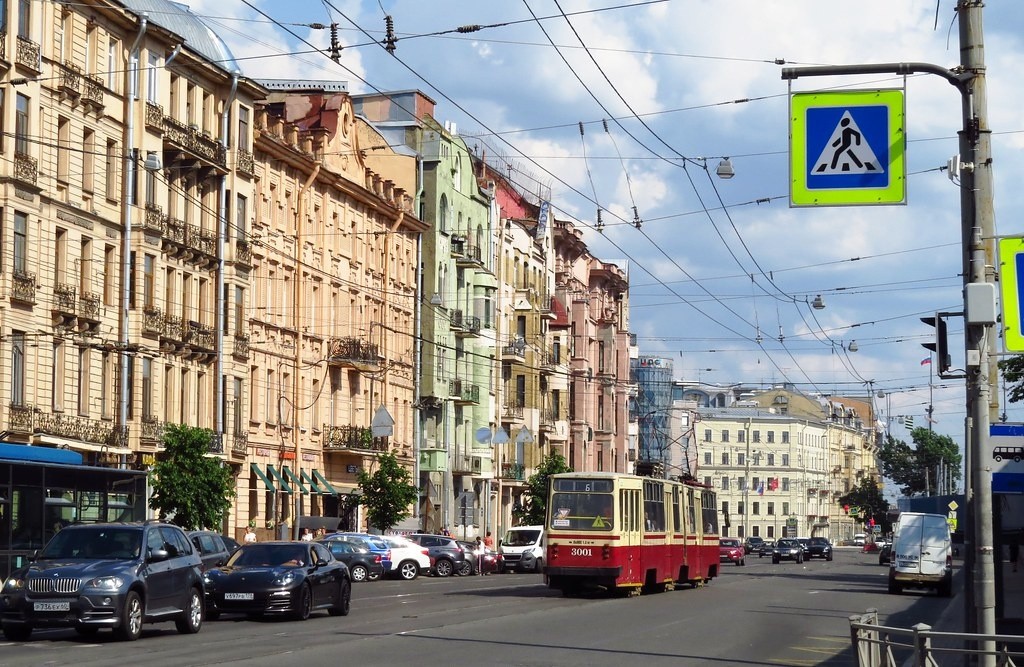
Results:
[792,93,908,207]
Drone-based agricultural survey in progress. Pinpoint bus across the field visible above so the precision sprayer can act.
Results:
[541,408,733,598]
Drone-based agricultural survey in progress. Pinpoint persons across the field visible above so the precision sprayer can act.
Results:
[438,527,450,536]
[287,549,318,567]
[126,534,150,558]
[300,528,312,541]
[475,536,486,576]
[485,531,493,550]
[243,526,256,544]
[645,510,652,530]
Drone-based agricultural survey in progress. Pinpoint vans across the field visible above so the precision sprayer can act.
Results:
[499,524,546,573]
[890,512,954,597]
[853,533,865,547]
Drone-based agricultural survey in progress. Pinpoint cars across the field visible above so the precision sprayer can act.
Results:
[153,530,242,577]
[879,544,892,565]
[313,540,384,583]
[719,537,746,566]
[804,537,835,560]
[455,540,507,576]
[205,540,352,621]
[744,537,766,555]
[798,538,810,556]
[762,541,776,556]
[378,535,431,580]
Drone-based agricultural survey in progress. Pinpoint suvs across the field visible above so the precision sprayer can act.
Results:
[399,533,465,578]
[1,519,210,642]
[772,537,805,564]
[315,532,393,575]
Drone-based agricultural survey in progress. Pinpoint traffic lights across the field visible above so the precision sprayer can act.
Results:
[920,315,947,378]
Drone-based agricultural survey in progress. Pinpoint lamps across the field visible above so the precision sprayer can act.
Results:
[848,340,858,352]
[144,153,160,169]
[877,390,885,398]
[716,157,735,179]
[812,294,826,309]
[898,416,904,424]
[431,293,442,305]
